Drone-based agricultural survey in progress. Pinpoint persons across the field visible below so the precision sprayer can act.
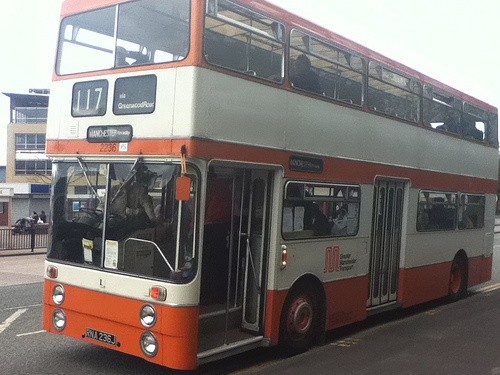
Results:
[200,174,232,306]
[39,210,47,223]
[32,211,39,224]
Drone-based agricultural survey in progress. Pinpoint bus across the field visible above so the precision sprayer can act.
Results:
[42,0,500,370]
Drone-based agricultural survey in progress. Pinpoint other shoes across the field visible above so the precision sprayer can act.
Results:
[213,297,224,303]
